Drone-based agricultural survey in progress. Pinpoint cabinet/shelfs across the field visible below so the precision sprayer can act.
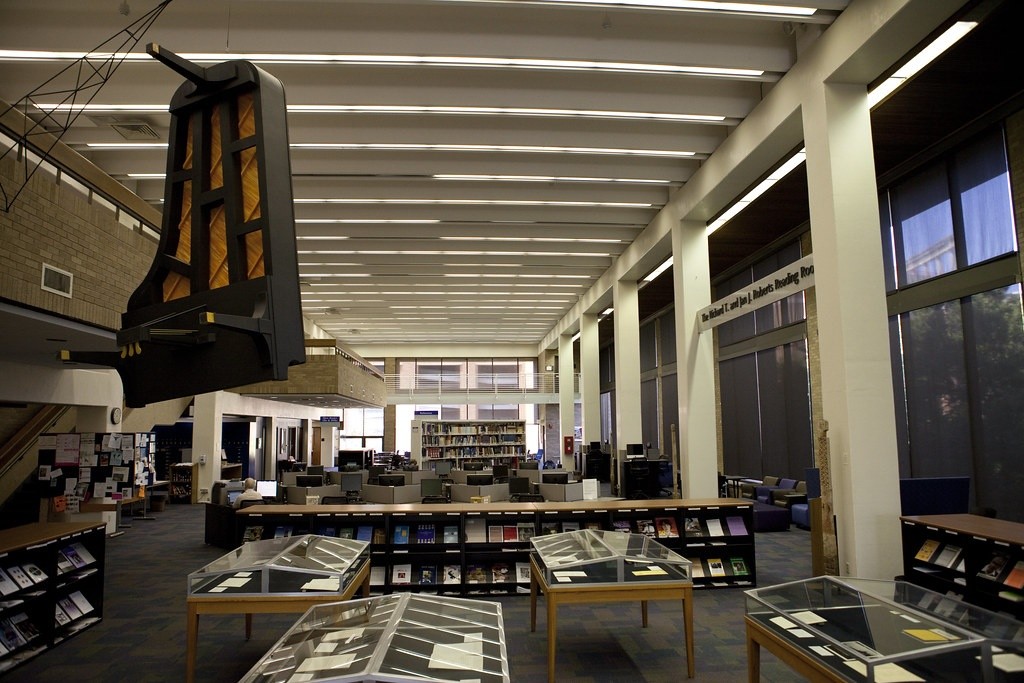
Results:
[900,514,1024,648]
[410,419,527,470]
[235,497,757,597]
[168,462,194,505]
[0,522,107,677]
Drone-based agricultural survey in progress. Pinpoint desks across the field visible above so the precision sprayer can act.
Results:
[80,496,156,527]
[722,476,750,498]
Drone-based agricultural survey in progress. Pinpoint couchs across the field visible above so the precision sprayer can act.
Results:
[742,476,811,530]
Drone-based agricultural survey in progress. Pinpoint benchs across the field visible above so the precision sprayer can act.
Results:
[741,498,791,533]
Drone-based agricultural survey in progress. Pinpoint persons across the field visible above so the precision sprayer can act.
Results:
[659,519,677,536]
[232,478,262,508]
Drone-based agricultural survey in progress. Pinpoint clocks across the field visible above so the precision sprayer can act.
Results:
[110,407,122,425]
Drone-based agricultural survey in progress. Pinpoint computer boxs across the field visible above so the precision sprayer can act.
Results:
[532,482,539,494]
[446,486,451,503]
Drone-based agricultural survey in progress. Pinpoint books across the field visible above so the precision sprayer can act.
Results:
[902,539,1024,672]
[247,518,749,584]
[423,423,524,465]
[0,544,99,655]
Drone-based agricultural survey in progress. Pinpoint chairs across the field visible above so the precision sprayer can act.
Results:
[240,500,267,509]
[421,497,449,504]
[628,454,673,500]
[321,496,348,505]
[509,493,545,502]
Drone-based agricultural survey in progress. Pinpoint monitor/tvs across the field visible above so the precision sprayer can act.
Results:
[227,490,244,504]
[590,442,600,449]
[292,463,568,498]
[627,444,644,459]
[255,480,278,498]
